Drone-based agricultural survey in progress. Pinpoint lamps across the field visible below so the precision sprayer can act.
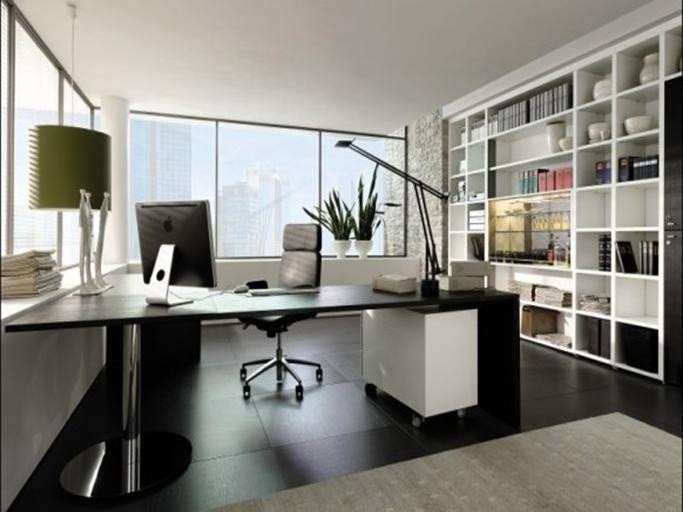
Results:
[332,137,453,301]
[28,2,114,212]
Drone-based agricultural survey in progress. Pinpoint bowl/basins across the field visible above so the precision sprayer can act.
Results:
[587,122,610,141]
[623,115,654,136]
[559,137,572,151]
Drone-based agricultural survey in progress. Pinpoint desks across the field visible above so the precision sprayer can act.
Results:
[5,273,519,503]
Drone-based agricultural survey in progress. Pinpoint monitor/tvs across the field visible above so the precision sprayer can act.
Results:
[135,199,217,306]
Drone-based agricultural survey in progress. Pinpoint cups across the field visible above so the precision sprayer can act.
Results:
[545,123,564,154]
[494,248,547,265]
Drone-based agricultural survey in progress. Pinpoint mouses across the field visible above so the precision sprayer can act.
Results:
[233,285,248,293]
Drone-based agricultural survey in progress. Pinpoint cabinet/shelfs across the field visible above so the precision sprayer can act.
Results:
[439,8,683,390]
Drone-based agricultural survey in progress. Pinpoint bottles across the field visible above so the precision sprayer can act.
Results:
[546,234,570,266]
[592,73,612,100]
[529,211,570,232]
[638,52,659,85]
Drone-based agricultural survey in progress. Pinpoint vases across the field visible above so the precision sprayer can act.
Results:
[546,51,659,153]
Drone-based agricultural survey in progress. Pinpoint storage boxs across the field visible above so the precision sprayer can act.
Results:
[521,304,558,338]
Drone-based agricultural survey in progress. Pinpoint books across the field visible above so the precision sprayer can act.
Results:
[460,83,573,144]
[519,155,659,194]
[615,240,658,275]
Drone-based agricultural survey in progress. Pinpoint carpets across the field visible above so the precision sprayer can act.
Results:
[203,409,683,511]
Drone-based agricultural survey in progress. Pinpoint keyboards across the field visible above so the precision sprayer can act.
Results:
[249,288,318,295]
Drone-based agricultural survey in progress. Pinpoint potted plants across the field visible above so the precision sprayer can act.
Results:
[302,189,359,259]
[338,160,402,258]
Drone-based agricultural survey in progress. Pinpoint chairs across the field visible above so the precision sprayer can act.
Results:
[228,223,324,403]
